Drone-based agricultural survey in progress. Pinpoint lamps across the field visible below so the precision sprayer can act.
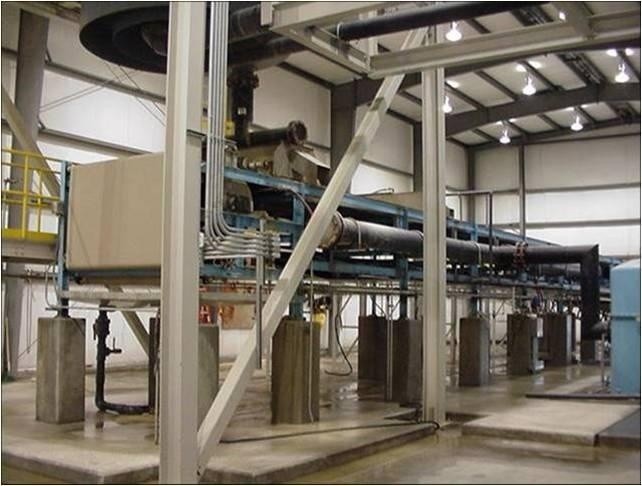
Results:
[445,21,463,41]
[571,116,583,131]
[614,64,630,83]
[522,76,536,96]
[441,96,453,113]
[499,130,511,144]
